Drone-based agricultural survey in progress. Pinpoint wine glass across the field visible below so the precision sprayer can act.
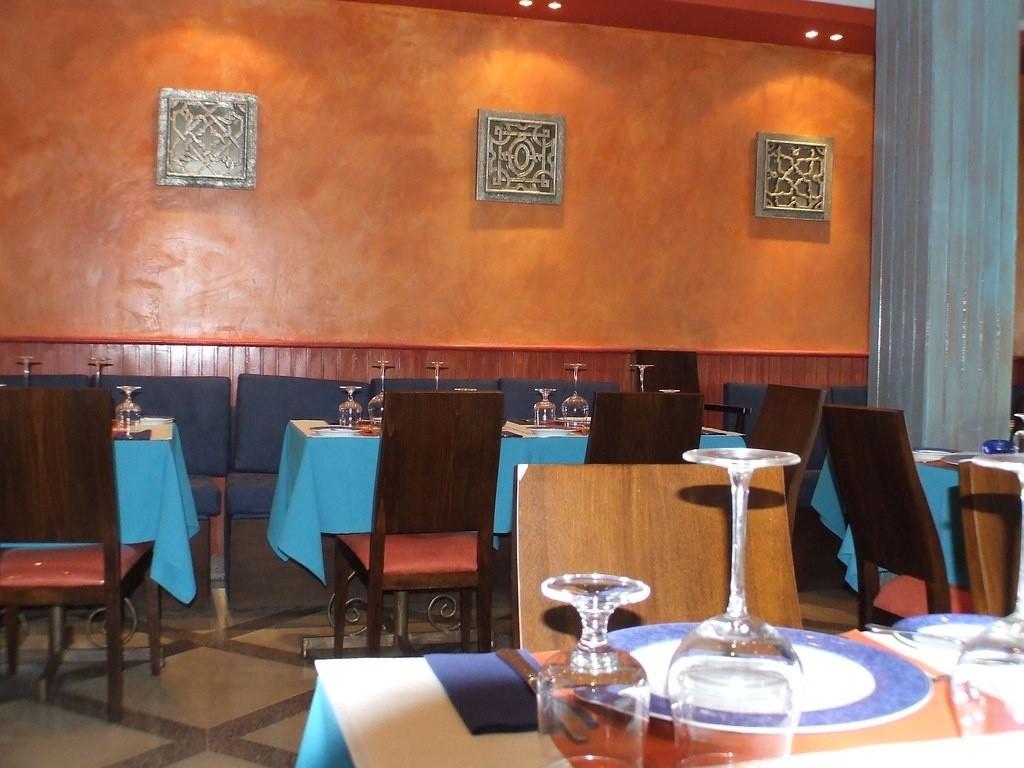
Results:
[533,389,557,422]
[536,573,650,768]
[86,357,111,388]
[659,389,680,393]
[115,386,141,427]
[665,448,802,766]
[1012,413,1024,458]
[629,364,654,391]
[949,453,1023,766]
[561,364,589,424]
[367,361,395,426]
[426,361,447,390]
[14,355,39,387]
[338,386,363,426]
[454,388,478,392]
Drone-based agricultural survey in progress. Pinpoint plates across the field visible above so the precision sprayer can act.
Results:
[308,426,362,436]
[568,619,933,732]
[140,418,173,424]
[892,613,1004,654]
[558,417,592,424]
[940,455,979,466]
[526,426,580,438]
[911,447,962,456]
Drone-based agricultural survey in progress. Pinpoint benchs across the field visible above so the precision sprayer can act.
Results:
[0,374,231,622]
[724,383,1023,588]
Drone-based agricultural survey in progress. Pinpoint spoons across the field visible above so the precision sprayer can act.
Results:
[867,622,1024,666]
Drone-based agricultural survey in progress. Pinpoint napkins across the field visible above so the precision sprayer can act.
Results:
[326,417,340,424]
[424,647,599,733]
[702,430,726,435]
[501,431,522,438]
[114,430,151,440]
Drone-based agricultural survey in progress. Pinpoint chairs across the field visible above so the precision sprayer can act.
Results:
[225,373,621,610]
[749,384,1024,634]
[512,463,802,653]
[0,389,162,725]
[635,349,978,629]
[494,392,704,604]
[333,390,503,658]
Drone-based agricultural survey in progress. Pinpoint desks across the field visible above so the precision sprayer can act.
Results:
[295,635,1023,768]
[267,420,747,661]
[811,447,969,593]
[0,420,201,605]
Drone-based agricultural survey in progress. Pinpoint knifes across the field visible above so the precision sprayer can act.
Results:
[494,648,590,741]
